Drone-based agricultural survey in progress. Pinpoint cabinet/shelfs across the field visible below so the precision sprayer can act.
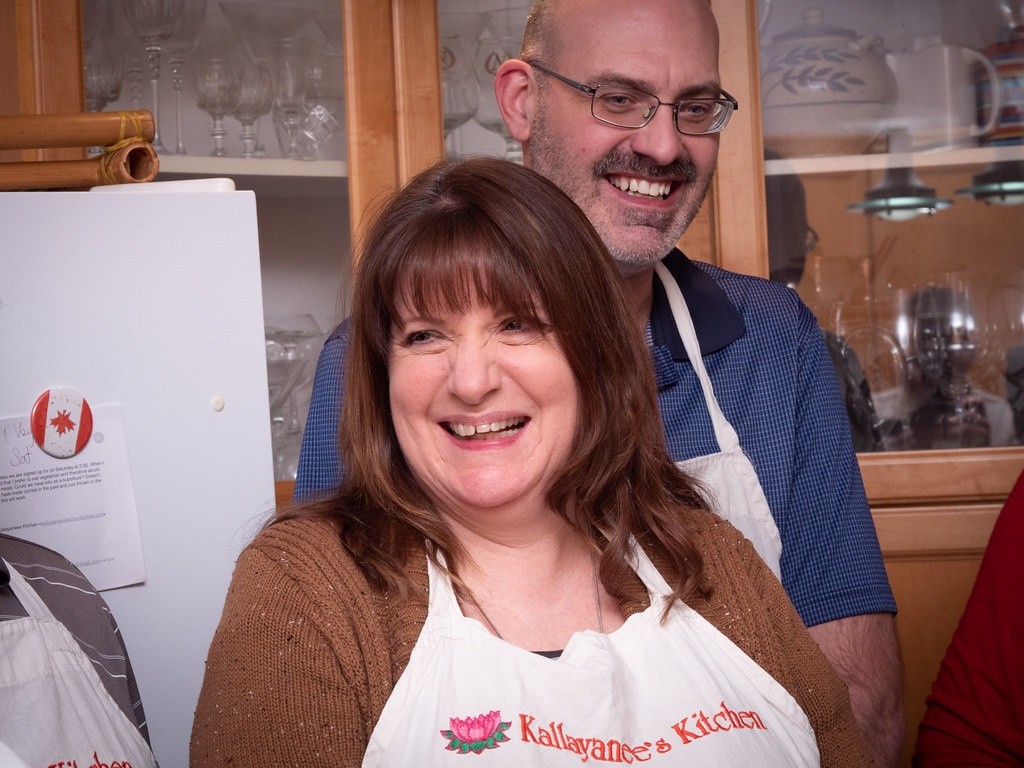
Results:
[0,2,1024,767]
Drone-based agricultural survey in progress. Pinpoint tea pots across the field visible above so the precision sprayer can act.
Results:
[756,9,894,162]
[875,32,1003,155]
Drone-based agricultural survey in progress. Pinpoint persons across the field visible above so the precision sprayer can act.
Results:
[290,1,908,768]
[0,531,163,768]
[187,154,872,767]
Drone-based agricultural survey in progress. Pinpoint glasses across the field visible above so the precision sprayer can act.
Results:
[527,62,738,136]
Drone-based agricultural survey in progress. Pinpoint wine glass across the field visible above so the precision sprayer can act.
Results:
[773,241,1024,451]
[73,0,348,160]
[261,306,324,480]
[433,0,534,171]
